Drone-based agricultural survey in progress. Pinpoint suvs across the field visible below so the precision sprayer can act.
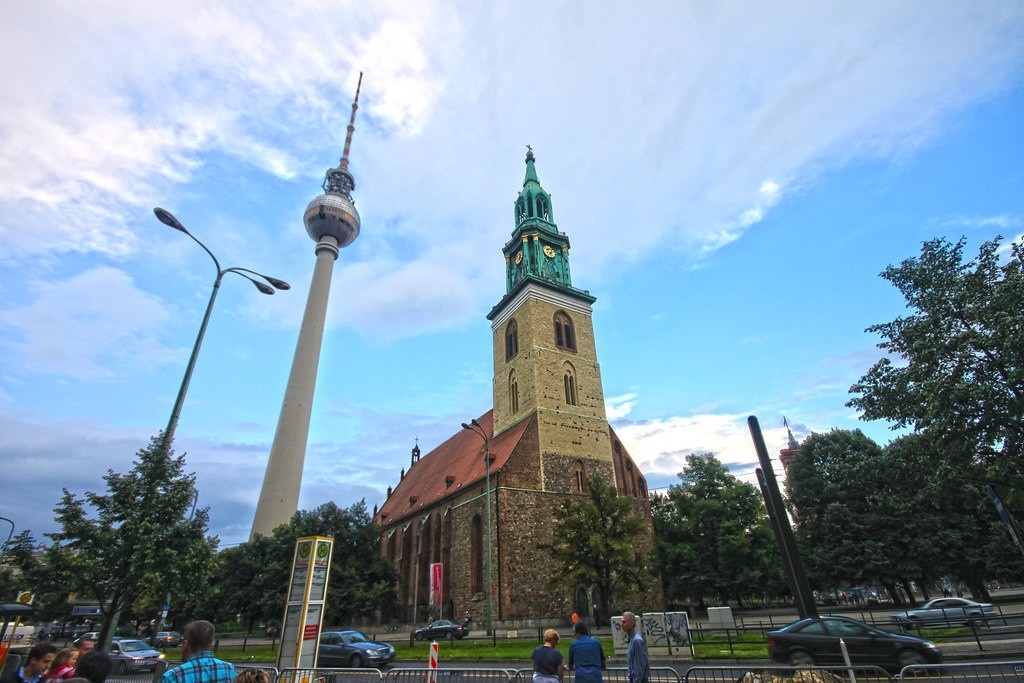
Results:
[145,631,182,648]
[317,630,396,669]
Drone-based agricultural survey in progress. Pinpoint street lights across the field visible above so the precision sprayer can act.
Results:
[91,207,291,661]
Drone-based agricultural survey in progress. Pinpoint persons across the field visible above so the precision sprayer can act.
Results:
[572,611,579,629]
[234,668,268,683]
[621,611,650,683]
[428,615,433,625]
[842,593,858,604]
[158,620,237,683]
[0,643,55,683]
[872,591,876,599]
[532,628,564,683]
[593,604,601,630]
[464,611,472,626]
[47,647,79,680]
[943,587,953,597]
[568,621,606,683]
[76,637,96,656]
[65,651,112,683]
[956,587,962,598]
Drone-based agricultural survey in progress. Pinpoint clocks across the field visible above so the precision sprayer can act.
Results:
[543,245,556,259]
[515,250,524,264]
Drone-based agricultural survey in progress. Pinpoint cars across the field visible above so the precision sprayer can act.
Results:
[413,619,469,642]
[765,614,943,676]
[888,596,995,631]
[87,639,166,677]
[37,617,105,642]
[72,631,123,650]
